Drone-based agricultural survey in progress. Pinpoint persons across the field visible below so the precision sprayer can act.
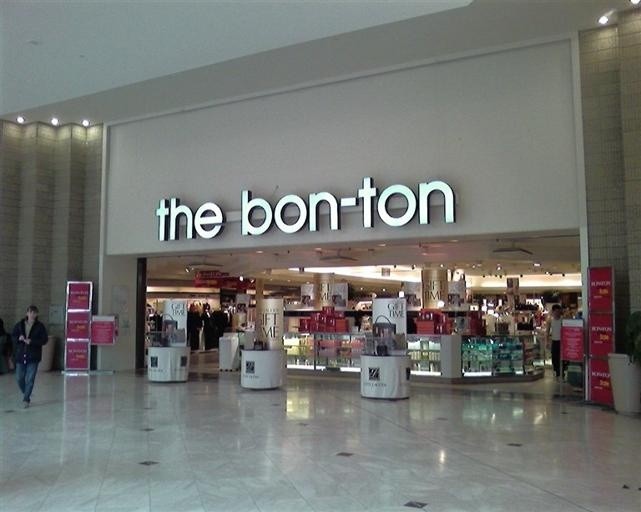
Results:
[0,318,9,375]
[237,303,247,313]
[302,295,311,304]
[187,303,229,353]
[332,295,342,305]
[11,305,48,408]
[544,303,564,377]
[153,312,162,330]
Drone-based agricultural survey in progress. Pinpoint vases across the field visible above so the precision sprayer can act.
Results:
[36,334,56,375]
[607,351,640,419]
